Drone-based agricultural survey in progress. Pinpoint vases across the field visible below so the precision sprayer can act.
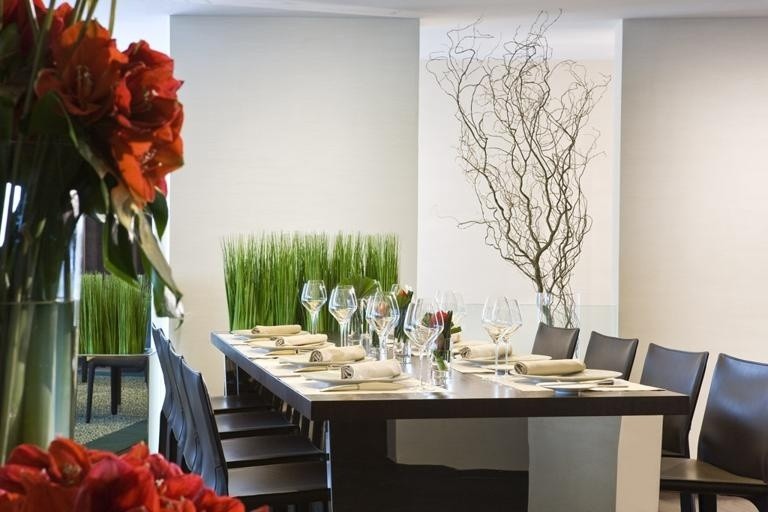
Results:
[1,301,76,448]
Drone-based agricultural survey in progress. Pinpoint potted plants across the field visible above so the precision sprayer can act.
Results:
[77,272,150,368]
[225,237,275,337]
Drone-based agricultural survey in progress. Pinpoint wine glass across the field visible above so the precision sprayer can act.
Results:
[300,279,523,388]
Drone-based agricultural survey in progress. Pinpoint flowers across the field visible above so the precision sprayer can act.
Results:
[0,0,184,299]
[2,435,273,512]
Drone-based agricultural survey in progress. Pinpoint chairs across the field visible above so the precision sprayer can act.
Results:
[530,323,580,359]
[660,353,767,512]
[583,332,639,380]
[147,321,339,511]
[641,343,709,511]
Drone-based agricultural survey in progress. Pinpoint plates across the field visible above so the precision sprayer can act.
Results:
[228,328,411,385]
[467,351,630,381]
